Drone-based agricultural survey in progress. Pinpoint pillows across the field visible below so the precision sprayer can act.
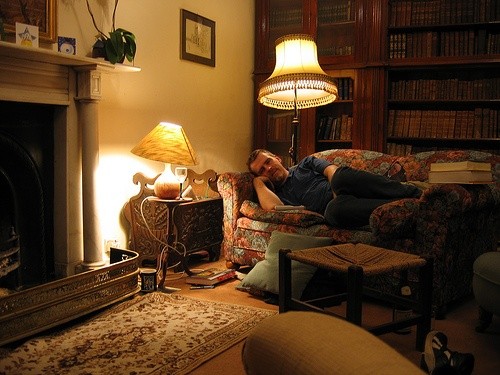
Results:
[242,231,334,302]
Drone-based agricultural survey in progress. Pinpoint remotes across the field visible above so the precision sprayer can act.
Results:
[274,204,306,210]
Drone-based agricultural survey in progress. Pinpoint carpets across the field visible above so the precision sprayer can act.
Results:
[0,290,277,375]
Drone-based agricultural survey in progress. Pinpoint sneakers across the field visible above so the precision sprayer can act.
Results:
[425,330,476,375]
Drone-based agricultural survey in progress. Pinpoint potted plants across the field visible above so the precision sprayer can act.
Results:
[85,0,136,64]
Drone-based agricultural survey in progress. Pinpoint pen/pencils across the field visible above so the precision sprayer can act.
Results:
[190,285,214,290]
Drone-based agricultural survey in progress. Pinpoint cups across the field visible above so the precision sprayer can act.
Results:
[140,269,158,292]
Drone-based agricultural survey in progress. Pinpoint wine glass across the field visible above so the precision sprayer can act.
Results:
[174,167,188,201]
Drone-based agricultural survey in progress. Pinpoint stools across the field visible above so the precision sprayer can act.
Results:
[279,239,432,352]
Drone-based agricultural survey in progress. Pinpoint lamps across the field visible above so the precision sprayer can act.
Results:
[131,121,199,198]
[257,33,339,167]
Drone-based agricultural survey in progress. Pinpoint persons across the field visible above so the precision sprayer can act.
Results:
[247,149,430,230]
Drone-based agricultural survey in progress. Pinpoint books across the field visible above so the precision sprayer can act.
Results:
[274,206,305,211]
[265,0,500,170]
[185,268,236,286]
[428,160,495,184]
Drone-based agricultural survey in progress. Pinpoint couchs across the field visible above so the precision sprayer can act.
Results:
[217,149,500,322]
[239,311,427,375]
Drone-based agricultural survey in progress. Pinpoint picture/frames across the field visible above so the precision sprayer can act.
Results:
[0,0,57,43]
[180,9,216,67]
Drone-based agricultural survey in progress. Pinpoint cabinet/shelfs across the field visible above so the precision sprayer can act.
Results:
[123,169,224,273]
[251,0,500,168]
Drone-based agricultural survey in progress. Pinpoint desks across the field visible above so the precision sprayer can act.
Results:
[147,196,194,291]
